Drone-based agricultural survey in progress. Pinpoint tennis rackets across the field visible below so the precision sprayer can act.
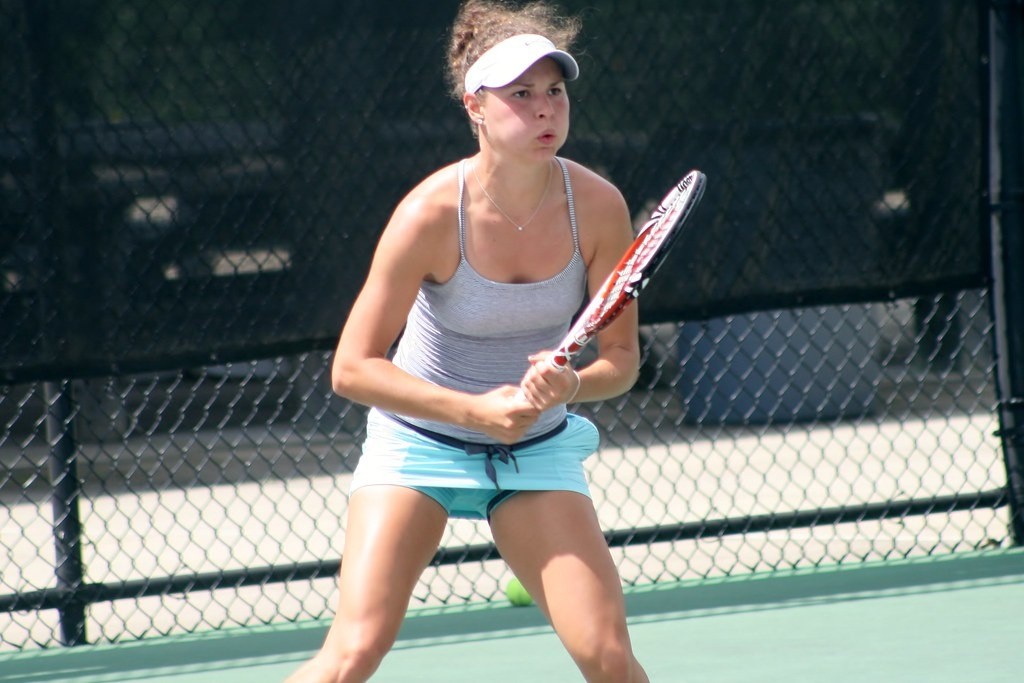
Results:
[514,168,708,407]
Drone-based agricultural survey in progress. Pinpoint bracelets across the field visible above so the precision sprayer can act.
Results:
[563,369,581,407]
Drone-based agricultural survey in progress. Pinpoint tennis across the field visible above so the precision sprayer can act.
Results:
[506,576,533,606]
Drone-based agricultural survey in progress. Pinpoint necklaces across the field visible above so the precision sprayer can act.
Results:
[472,158,553,230]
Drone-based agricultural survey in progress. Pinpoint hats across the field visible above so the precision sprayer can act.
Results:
[465,33,580,94]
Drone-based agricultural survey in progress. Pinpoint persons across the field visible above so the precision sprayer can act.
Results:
[280,1,654,683]
